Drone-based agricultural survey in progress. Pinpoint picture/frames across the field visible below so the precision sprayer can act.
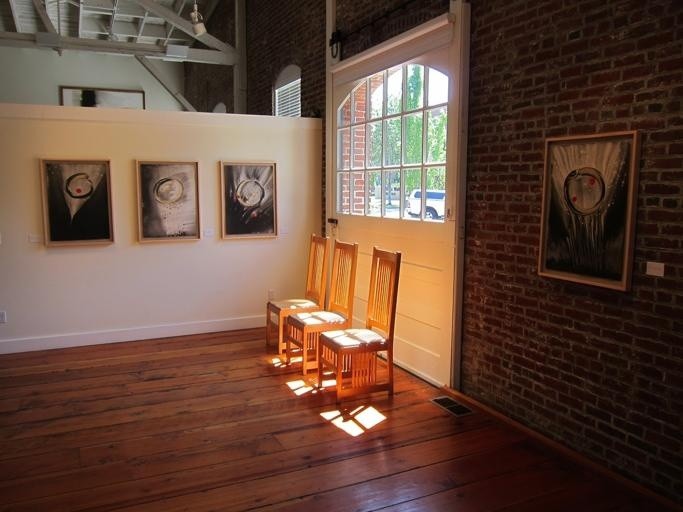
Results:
[56,85,146,110]
[37,157,278,249]
[534,129,642,293]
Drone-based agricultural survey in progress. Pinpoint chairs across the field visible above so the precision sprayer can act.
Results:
[266,233,404,405]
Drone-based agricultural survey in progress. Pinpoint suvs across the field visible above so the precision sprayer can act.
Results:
[386,185,399,199]
[406,188,445,220]
